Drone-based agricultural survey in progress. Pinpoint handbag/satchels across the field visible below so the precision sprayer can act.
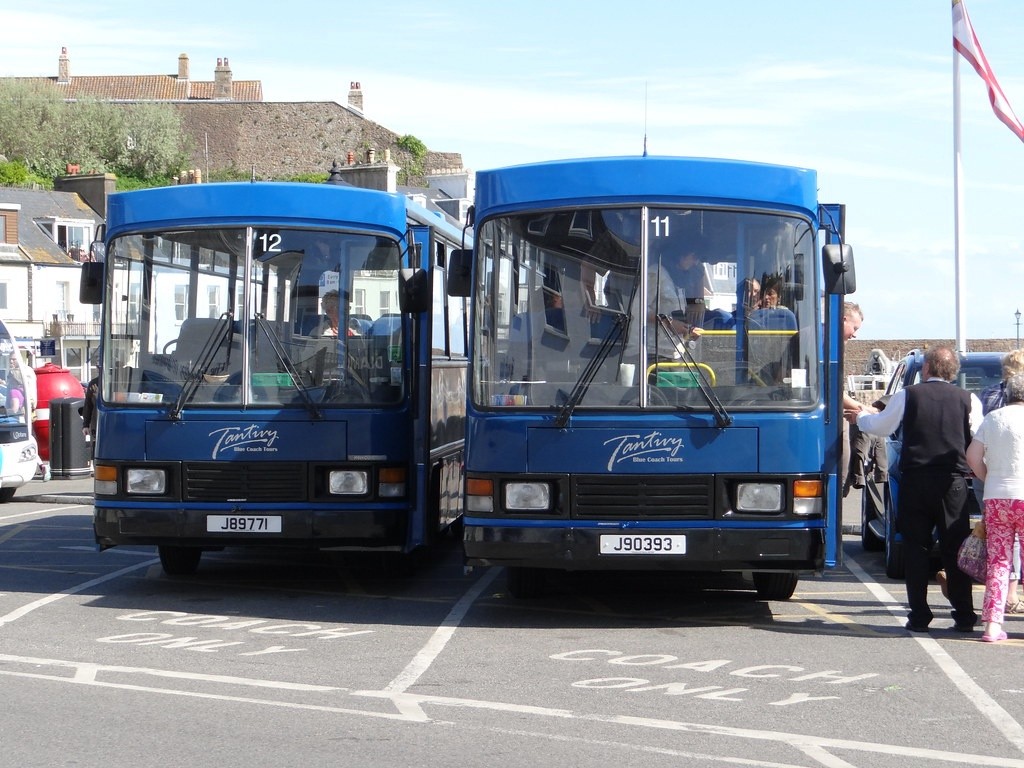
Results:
[958,509,987,583]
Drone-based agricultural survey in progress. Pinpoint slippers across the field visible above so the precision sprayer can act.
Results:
[981,631,1008,642]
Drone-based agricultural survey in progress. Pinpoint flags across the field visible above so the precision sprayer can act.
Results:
[952,0,1024,144]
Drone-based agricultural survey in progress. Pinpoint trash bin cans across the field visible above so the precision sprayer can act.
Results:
[48,396,93,479]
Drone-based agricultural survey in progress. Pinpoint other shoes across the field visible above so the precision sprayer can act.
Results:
[41,464,51,481]
[906,620,929,632]
[1005,597,1024,613]
[954,621,974,632]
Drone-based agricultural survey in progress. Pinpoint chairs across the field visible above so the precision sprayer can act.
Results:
[508,308,797,376]
[163,313,401,383]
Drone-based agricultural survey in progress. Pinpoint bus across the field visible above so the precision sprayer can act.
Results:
[0,318,40,501]
[78,180,521,577]
[446,153,860,603]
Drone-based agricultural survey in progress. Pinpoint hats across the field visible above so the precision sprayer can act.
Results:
[318,271,352,298]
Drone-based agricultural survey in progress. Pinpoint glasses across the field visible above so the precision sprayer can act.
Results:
[691,250,701,262]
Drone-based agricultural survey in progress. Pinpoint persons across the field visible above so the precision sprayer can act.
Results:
[748,303,880,414]
[732,276,790,321]
[936,348,1024,642]
[843,347,984,633]
[0,349,51,482]
[505,227,714,388]
[82,357,124,469]
[303,290,364,335]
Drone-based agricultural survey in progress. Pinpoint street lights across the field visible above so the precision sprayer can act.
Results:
[1014,309,1021,350]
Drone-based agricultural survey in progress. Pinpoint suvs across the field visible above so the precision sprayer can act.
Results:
[858,349,1017,580]
